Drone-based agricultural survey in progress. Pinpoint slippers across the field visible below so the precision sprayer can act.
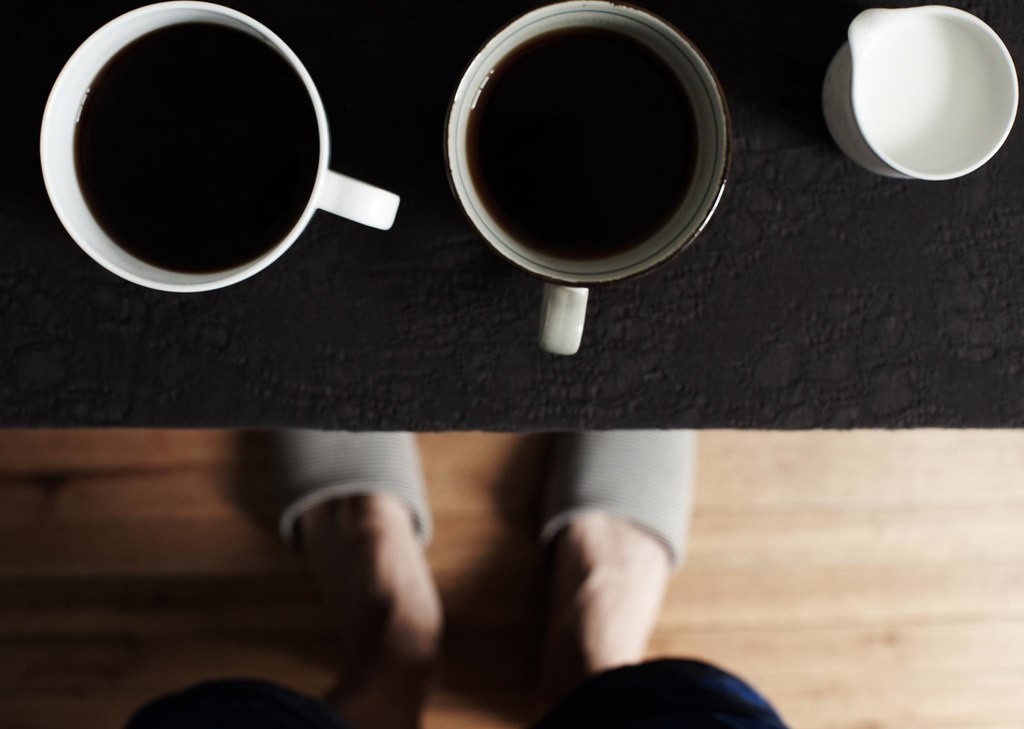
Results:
[536,428,694,573]
[265,428,433,554]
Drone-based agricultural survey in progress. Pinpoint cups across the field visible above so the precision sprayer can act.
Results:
[38,0,401,295]
[447,0,732,356]
[822,5,1020,181]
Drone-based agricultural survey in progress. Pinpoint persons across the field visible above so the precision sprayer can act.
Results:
[126,428,788,729]
